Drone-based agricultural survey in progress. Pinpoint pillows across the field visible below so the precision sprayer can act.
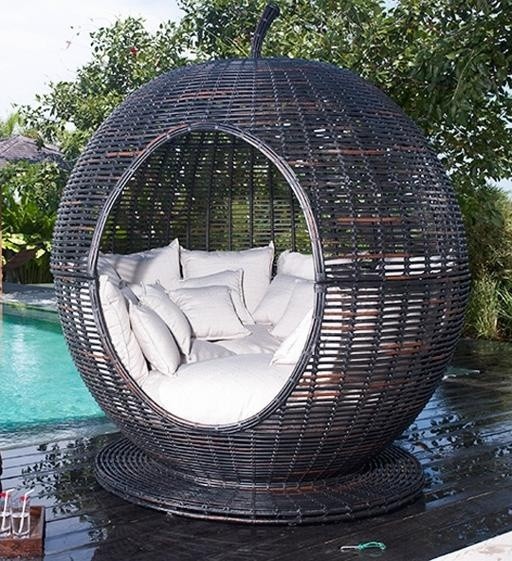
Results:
[98,236,317,384]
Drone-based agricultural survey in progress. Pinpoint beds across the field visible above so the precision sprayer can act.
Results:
[48,3,473,528]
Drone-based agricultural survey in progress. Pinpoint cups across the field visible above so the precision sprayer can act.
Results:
[0,496,32,537]
